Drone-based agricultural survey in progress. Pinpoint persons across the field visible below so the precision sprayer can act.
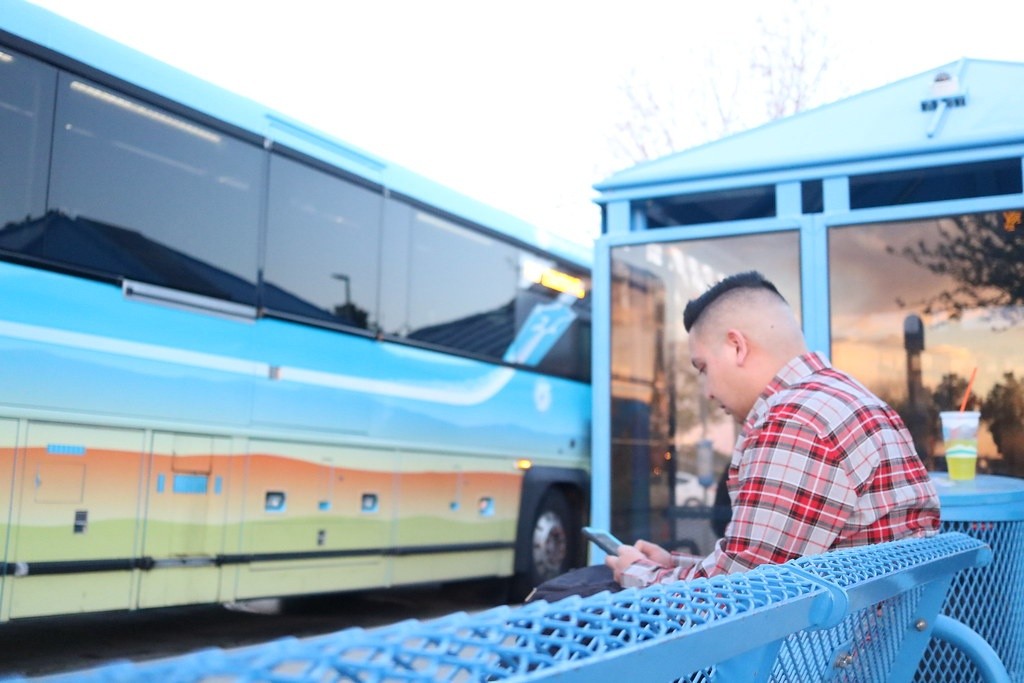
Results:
[475,270,941,682]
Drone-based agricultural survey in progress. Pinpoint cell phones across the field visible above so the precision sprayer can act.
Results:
[582,526,625,557]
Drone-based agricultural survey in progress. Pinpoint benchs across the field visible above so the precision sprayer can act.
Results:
[0,529,1011,683]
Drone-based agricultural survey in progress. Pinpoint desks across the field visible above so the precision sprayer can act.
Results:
[911,470,1024,683]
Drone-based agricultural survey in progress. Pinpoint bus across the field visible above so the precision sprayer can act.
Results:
[0,0,687,632]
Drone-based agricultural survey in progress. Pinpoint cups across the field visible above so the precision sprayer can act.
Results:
[939,412,981,479]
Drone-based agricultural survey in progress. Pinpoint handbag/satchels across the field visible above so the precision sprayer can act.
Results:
[528,566,622,601]
